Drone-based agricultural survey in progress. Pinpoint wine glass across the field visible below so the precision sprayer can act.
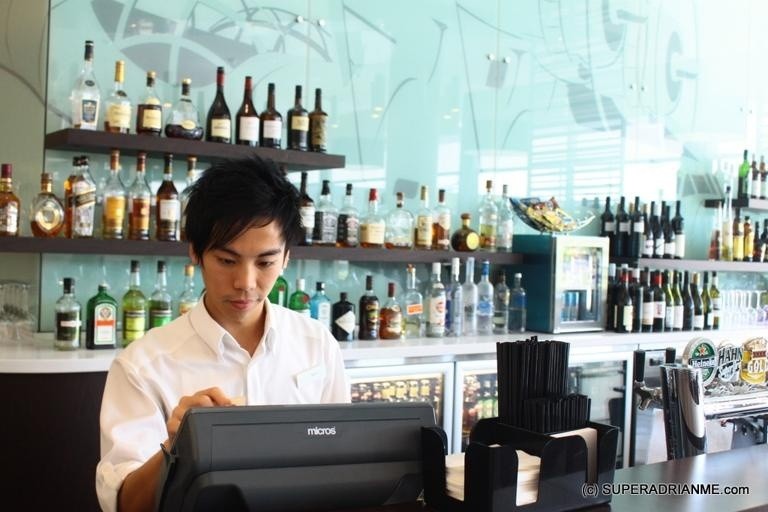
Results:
[719,289,768,327]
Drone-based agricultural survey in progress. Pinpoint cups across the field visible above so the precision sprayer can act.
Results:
[0,283,36,347]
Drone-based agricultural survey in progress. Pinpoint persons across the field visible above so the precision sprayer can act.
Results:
[95,150,352,512]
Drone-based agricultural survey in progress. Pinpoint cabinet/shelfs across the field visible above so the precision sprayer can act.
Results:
[343,342,454,456]
[454,339,634,468]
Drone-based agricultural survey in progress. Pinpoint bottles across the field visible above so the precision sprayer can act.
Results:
[492,269,511,333]
[722,186,735,259]
[497,185,514,248]
[55,279,82,348]
[414,186,435,248]
[206,66,232,143]
[287,82,309,150]
[600,196,615,265]
[259,83,285,150]
[712,273,726,328]
[477,259,496,332]
[88,285,115,348]
[354,381,447,423]
[446,257,464,336]
[179,264,199,315]
[618,194,631,264]
[461,257,480,333]
[383,191,416,248]
[182,155,196,236]
[268,274,288,305]
[759,156,768,198]
[752,220,762,241]
[333,290,355,341]
[737,148,754,197]
[315,180,339,245]
[104,59,132,133]
[1,164,22,234]
[311,87,329,152]
[359,273,380,340]
[29,173,65,238]
[631,194,646,264]
[607,268,710,332]
[297,170,318,245]
[337,183,361,246]
[101,149,126,238]
[478,179,497,246]
[358,188,386,247]
[744,215,754,261]
[68,39,102,130]
[646,200,686,259]
[148,261,172,327]
[751,153,760,198]
[288,278,309,314]
[706,202,723,261]
[0,283,37,347]
[402,263,425,336]
[136,71,164,134]
[157,150,178,241]
[431,188,452,251]
[454,209,478,250]
[758,237,768,258]
[236,76,261,146]
[381,283,401,339]
[68,155,97,236]
[123,260,145,347]
[128,147,151,241]
[509,272,530,331]
[311,279,330,328]
[425,262,447,334]
[460,372,499,439]
[733,206,745,261]
[165,78,205,139]
